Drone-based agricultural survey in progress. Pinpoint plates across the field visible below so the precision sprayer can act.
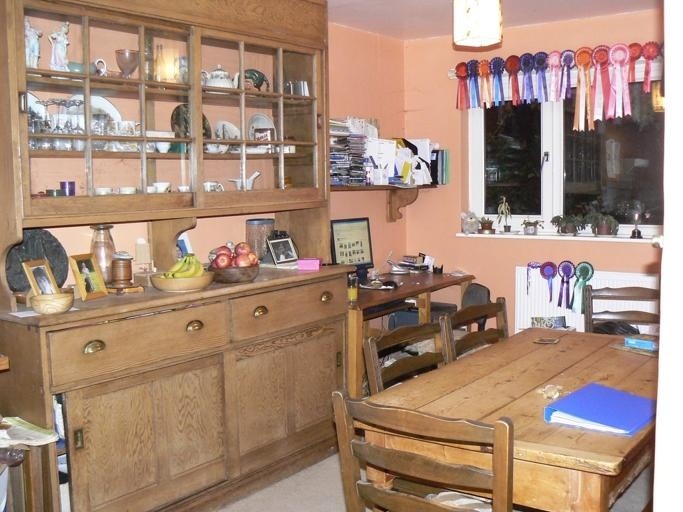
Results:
[246,113,277,144]
[169,103,212,142]
[214,119,241,140]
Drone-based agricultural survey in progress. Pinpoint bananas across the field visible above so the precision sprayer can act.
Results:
[162,253,204,279]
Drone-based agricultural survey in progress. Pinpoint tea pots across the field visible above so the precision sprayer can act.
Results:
[201,63,240,95]
[203,181,225,192]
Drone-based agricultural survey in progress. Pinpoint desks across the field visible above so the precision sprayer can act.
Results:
[330,327,658,512]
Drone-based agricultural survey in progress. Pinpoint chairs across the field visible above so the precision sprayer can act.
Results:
[581,283,659,338]
[362,315,447,392]
[444,297,509,358]
[331,388,513,512]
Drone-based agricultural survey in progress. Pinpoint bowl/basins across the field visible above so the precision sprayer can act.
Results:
[29,292,72,317]
[149,270,215,292]
[208,263,258,283]
[203,143,229,154]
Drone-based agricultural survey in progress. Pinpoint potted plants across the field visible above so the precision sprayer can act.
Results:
[522,221,544,235]
[479,218,496,234]
[588,212,618,235]
[551,213,588,235]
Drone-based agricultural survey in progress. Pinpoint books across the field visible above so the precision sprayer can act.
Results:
[328,115,452,188]
[0,415,61,446]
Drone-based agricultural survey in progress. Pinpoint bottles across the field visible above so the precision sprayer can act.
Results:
[90,225,116,284]
[139,30,168,88]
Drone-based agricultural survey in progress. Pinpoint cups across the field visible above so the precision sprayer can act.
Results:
[112,120,141,152]
[145,131,175,153]
[94,182,190,195]
[44,181,76,196]
[114,49,141,78]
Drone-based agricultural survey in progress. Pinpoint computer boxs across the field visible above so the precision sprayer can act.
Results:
[395,302,457,329]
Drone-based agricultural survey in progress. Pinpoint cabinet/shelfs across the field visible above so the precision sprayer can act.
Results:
[352,270,475,389]
[567,94,603,218]
[0,0,345,512]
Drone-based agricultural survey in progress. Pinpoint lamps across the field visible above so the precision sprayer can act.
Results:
[453,0,502,46]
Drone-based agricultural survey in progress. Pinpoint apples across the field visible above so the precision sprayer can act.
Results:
[209,242,258,269]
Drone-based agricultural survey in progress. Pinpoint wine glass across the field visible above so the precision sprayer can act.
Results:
[27,96,86,150]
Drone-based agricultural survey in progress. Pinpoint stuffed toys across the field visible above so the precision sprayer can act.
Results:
[458,210,479,234]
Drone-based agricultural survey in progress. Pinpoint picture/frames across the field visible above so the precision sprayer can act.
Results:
[70,252,109,301]
[22,259,59,296]
[264,236,298,267]
[329,217,374,270]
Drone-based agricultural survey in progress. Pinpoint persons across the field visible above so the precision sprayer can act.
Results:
[25,17,42,72]
[46,18,74,76]
[32,267,53,294]
[274,241,291,261]
[80,261,96,293]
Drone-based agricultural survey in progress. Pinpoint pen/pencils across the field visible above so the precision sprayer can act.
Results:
[370,156,389,169]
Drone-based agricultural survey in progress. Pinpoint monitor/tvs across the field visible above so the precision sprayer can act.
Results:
[330,217,375,286]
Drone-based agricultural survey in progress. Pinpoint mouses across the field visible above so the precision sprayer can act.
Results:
[382,281,397,289]
